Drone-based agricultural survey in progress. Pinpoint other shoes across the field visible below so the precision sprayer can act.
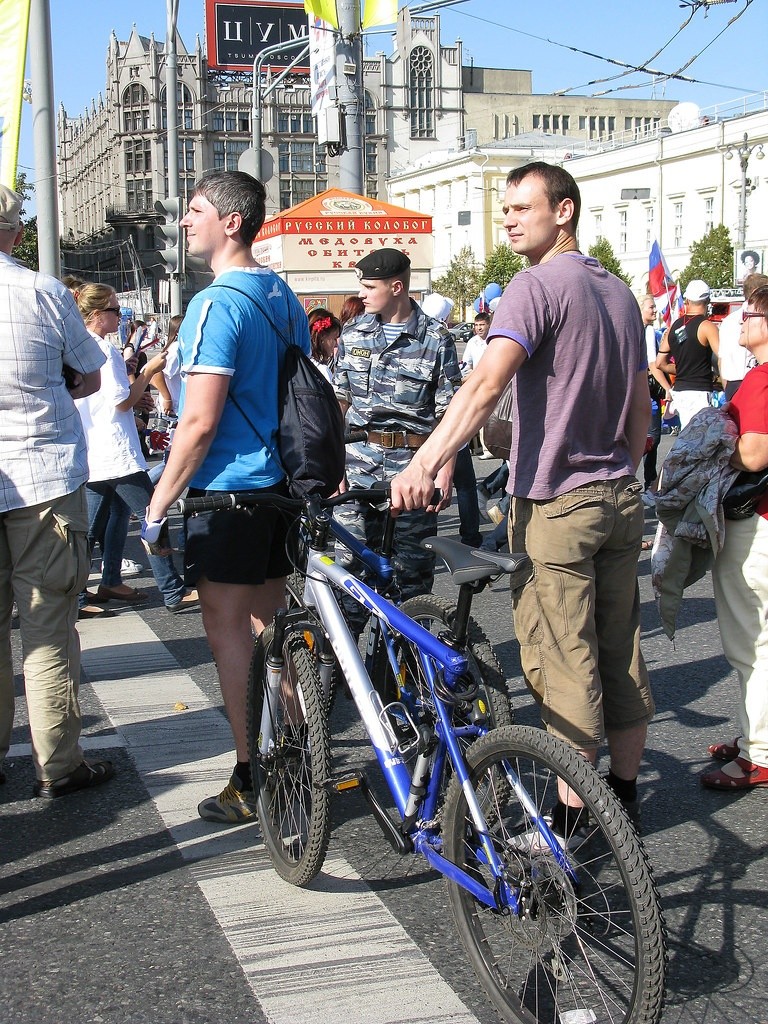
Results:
[78,604,115,619]
[79,591,110,603]
[477,450,495,460]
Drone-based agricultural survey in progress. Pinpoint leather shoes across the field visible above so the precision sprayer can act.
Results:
[165,599,201,614]
[98,585,149,600]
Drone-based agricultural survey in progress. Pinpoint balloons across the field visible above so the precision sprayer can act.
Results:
[474,282,503,314]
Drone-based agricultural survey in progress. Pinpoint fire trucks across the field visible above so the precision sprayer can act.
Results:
[679,287,752,328]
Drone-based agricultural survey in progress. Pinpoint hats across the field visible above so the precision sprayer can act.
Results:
[683,279,710,301]
[0,184,23,231]
[421,293,455,323]
[354,248,411,279]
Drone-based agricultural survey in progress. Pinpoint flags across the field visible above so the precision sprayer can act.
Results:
[648,239,685,328]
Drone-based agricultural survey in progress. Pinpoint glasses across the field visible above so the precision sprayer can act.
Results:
[102,305,121,316]
[742,309,765,320]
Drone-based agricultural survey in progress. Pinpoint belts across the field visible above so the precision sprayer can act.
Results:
[349,427,429,449]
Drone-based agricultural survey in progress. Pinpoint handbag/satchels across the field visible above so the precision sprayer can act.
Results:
[721,467,768,520]
[158,327,161,333]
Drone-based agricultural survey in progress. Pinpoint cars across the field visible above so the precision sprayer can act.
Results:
[447,323,475,342]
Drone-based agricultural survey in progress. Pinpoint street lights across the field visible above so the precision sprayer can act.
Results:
[723,132,768,250]
[77,230,102,283]
[153,199,178,273]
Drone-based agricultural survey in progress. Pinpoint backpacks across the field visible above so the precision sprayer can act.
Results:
[206,281,347,506]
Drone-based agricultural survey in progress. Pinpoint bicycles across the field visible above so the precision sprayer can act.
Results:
[180,489,674,1021]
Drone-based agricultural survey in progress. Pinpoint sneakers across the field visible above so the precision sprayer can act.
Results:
[101,558,144,576]
[588,778,643,836]
[641,492,656,510]
[498,805,594,867]
[474,482,489,521]
[198,763,293,824]
[264,753,314,783]
[487,506,504,527]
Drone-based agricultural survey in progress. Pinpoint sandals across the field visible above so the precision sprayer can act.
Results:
[641,540,654,550]
[709,735,743,760]
[700,757,768,788]
[33,757,114,798]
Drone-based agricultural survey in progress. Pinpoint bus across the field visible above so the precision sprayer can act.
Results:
[119,306,135,344]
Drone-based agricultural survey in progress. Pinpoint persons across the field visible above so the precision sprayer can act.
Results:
[421,294,514,554]
[142,168,313,825]
[55,276,201,620]
[307,296,365,385]
[700,275,768,790]
[390,161,651,857]
[0,182,115,801]
[738,250,760,280]
[329,249,462,686]
[636,272,768,554]
